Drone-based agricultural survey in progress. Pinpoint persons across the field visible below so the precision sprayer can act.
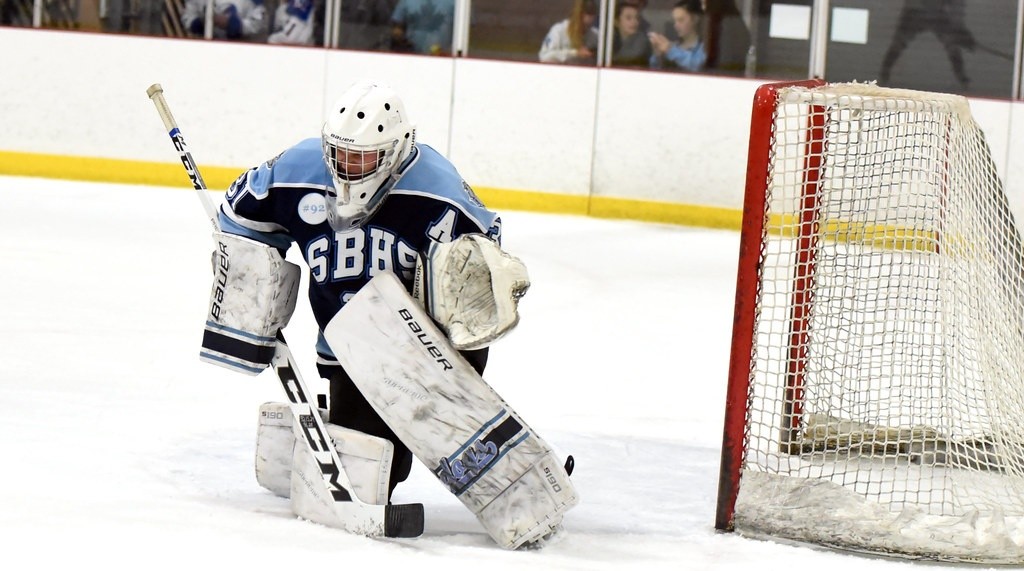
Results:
[197,84,530,536]
[876,0,975,91]
[387,0,453,55]
[181,0,319,48]
[537,0,752,79]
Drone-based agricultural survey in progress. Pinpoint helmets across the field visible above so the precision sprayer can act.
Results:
[321,89,420,234]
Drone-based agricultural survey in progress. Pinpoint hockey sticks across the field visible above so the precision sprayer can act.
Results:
[145,81,423,539]
